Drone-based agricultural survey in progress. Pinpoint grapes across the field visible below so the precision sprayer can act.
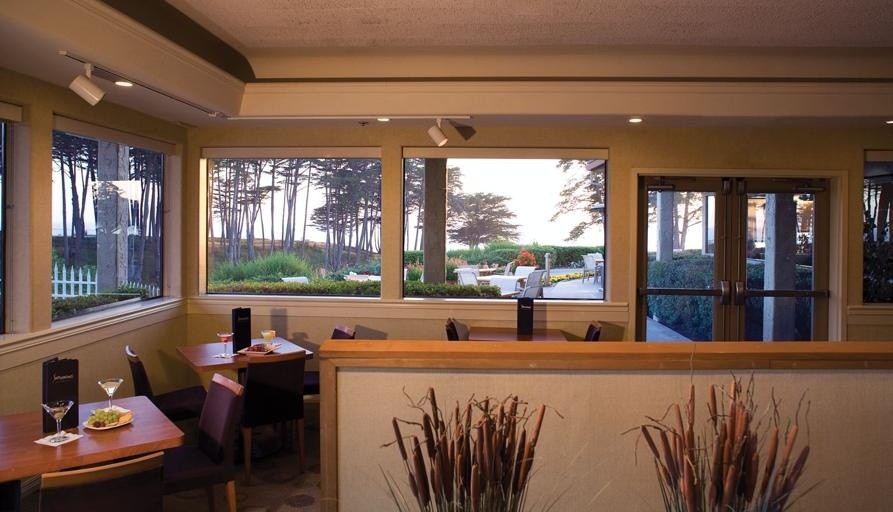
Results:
[87,409,119,427]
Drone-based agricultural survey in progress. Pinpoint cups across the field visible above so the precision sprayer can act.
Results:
[262,330,274,342]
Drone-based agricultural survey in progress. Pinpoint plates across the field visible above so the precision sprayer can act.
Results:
[237,343,282,356]
[84,419,133,431]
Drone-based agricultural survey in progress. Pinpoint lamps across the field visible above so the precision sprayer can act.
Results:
[426,118,450,150]
[69,62,106,108]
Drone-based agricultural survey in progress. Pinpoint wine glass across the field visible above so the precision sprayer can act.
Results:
[42,400,74,442]
[98,378,124,407]
[217,328,234,357]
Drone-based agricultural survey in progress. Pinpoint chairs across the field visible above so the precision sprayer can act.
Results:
[342,269,408,282]
[302,322,356,405]
[580,251,604,289]
[452,262,548,299]
[160,372,245,512]
[445,317,468,341]
[124,344,209,423]
[585,319,602,340]
[38,451,165,511]
[232,350,306,481]
